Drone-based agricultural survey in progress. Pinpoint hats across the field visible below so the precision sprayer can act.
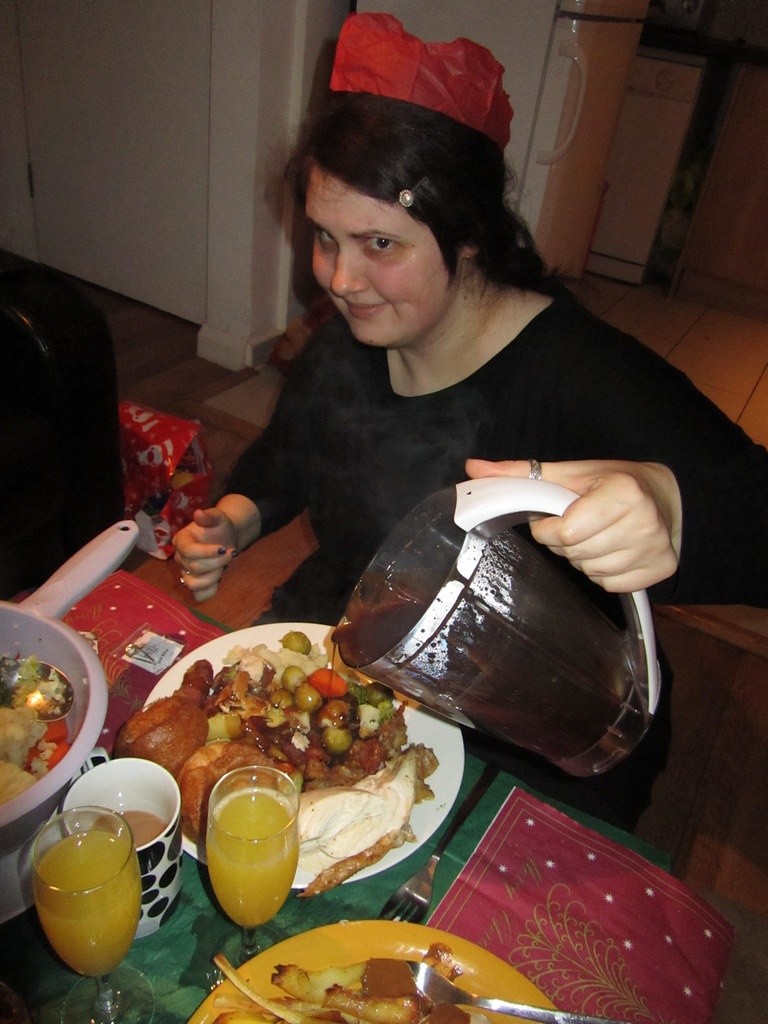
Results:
[330,11,514,152]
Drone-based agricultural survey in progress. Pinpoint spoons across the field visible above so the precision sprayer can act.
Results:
[0,656,75,722]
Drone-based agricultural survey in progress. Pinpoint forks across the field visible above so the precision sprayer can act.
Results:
[362,959,629,1024]
[378,764,500,924]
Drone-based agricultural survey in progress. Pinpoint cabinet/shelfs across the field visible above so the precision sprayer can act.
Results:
[0,0,352,372]
[664,55,768,325]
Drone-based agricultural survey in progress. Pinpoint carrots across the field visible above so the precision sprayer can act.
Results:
[307,667,348,698]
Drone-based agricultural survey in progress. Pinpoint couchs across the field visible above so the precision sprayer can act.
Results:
[0,249,123,593]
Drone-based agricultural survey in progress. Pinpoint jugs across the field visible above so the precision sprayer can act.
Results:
[331,475,662,779]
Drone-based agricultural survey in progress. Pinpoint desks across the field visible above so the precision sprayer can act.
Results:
[0,570,768,1024]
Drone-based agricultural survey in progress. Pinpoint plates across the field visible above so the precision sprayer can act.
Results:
[142,623,465,891]
[0,818,60,926]
[185,919,559,1024]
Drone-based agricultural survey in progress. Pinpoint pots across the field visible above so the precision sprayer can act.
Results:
[0,520,141,859]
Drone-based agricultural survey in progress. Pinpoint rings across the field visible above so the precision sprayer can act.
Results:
[180,571,190,584]
[528,459,541,480]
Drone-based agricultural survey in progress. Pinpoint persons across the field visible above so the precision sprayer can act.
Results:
[170,12,768,837]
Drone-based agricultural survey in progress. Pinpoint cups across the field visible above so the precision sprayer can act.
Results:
[62,756,183,940]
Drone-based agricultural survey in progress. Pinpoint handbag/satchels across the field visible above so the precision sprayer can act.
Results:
[118,400,216,559]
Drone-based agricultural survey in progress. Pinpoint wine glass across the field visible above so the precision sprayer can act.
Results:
[31,804,156,1024]
[207,765,301,971]
